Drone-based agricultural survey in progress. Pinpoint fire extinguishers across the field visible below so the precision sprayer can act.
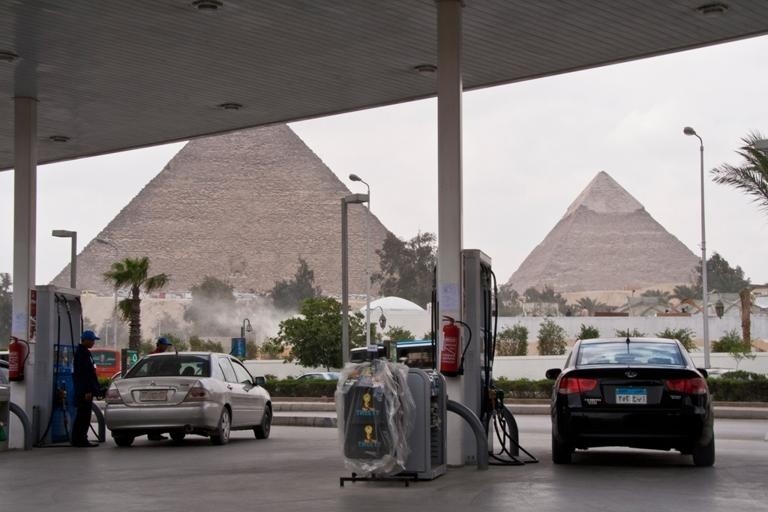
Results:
[441,316,472,377]
[9,335,30,382]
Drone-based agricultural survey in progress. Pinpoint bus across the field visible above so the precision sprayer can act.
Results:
[352,340,435,370]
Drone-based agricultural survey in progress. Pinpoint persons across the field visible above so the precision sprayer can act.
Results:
[71,330,102,447]
[147,336,173,440]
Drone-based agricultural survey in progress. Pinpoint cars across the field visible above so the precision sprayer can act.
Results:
[547,332,716,467]
[104,350,274,448]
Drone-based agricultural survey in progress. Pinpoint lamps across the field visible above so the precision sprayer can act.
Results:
[50,136,70,141]
[2,50,20,64]
[218,103,242,112]
[191,1,223,12]
[698,3,729,18]
[412,63,436,78]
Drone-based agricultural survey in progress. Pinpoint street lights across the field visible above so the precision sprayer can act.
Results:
[242,318,251,335]
[684,124,724,368]
[350,170,376,350]
[94,237,120,351]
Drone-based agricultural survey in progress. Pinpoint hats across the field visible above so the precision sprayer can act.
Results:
[81,330,100,339]
[156,338,172,345]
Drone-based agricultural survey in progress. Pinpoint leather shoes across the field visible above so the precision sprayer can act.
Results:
[73,437,99,446]
[147,434,168,440]
